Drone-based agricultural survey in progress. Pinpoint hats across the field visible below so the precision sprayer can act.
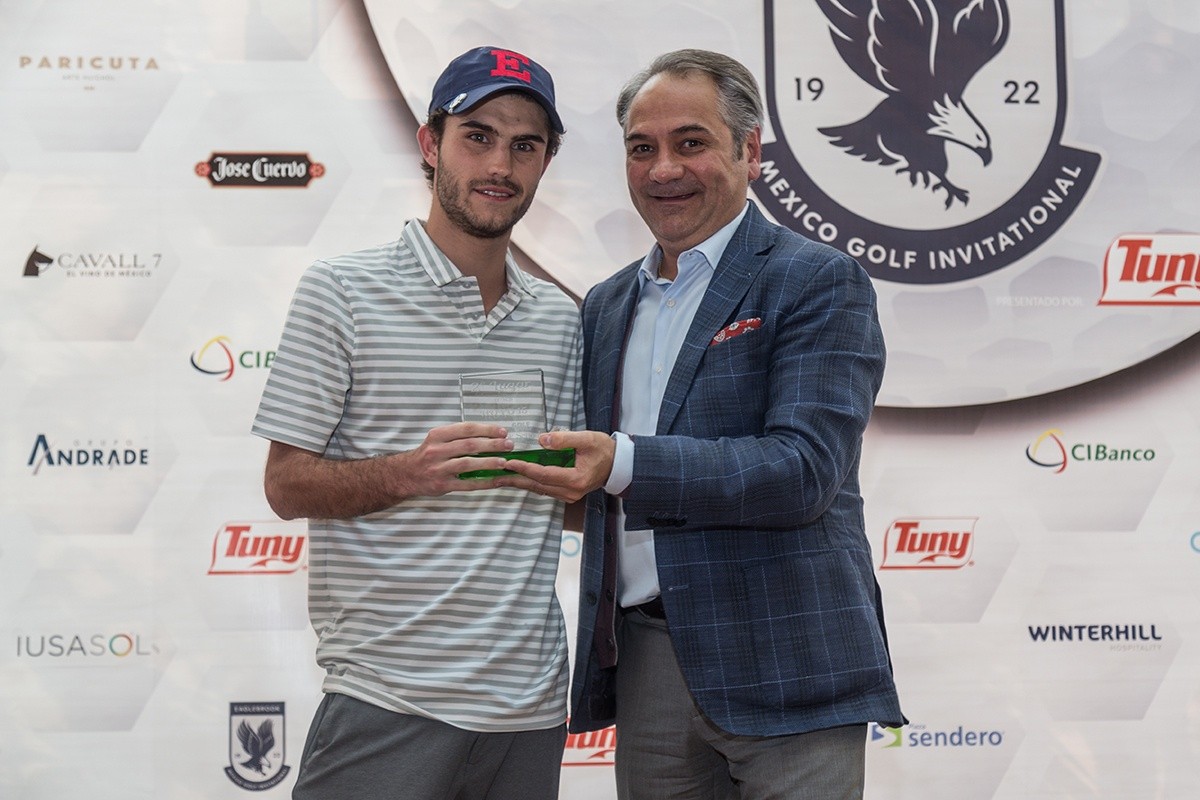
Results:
[429,46,566,135]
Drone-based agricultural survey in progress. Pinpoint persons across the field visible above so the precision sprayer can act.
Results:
[251,46,584,800]
[496,49,910,800]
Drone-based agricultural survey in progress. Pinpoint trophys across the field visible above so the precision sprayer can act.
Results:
[455,368,576,481]
